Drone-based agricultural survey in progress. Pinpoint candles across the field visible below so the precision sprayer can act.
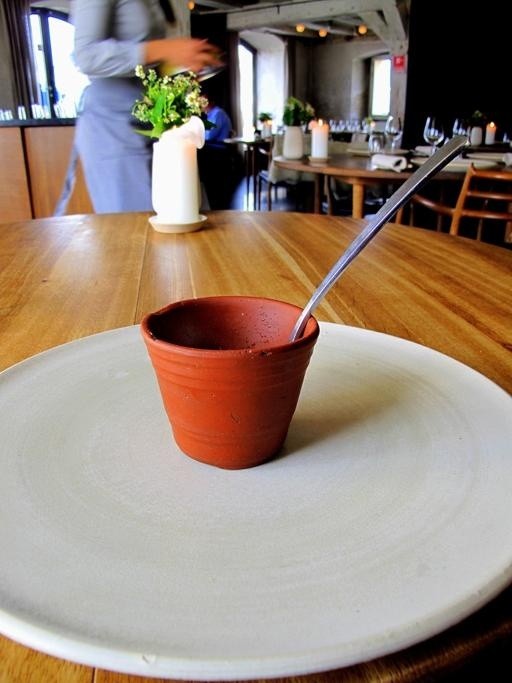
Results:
[484,121,496,144]
[265,118,273,136]
[309,118,330,159]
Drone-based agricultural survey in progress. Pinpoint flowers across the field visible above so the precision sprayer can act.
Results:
[257,111,271,123]
[282,97,316,125]
[130,63,208,140]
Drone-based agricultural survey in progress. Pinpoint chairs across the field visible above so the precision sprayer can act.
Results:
[222,126,415,225]
[394,161,511,246]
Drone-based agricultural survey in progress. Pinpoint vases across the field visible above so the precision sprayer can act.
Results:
[151,114,206,222]
[470,127,484,148]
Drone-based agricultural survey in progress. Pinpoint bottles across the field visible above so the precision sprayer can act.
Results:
[60,93,76,118]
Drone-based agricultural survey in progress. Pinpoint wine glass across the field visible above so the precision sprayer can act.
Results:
[368,115,469,155]
[328,118,368,133]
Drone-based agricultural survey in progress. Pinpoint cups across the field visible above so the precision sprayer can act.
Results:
[0,103,66,120]
[470,127,482,146]
[484,122,497,145]
[137,296,321,467]
[311,123,329,158]
[264,120,273,135]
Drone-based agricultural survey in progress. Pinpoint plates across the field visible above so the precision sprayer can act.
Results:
[411,157,498,171]
[467,151,505,161]
[148,212,209,233]
[307,155,332,164]
[346,146,409,157]
[2,322,512,682]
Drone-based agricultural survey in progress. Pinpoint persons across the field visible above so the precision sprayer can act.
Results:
[70,0,213,214]
[198,88,234,150]
